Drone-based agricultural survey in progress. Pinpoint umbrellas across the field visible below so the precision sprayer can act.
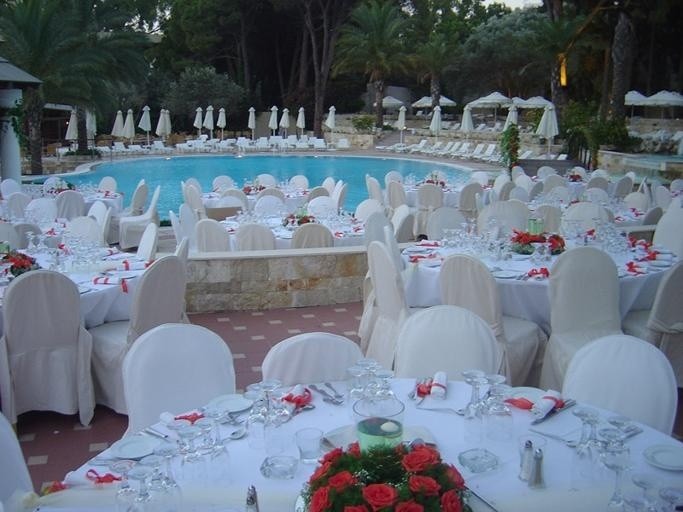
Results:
[216,107,227,141]
[324,106,336,150]
[247,107,256,141]
[372,91,560,157]
[64,106,172,152]
[202,105,214,139]
[279,108,290,138]
[296,106,306,135]
[268,106,278,136]
[623,89,683,120]
[193,107,203,136]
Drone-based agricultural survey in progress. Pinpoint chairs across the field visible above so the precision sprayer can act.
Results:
[375,139,569,164]
[624,126,683,151]
[0,268,97,428]
[168,173,349,253]
[0,174,189,330]
[357,167,683,394]
[0,413,35,512]
[84,254,188,416]
[121,322,236,442]
[261,332,365,387]
[561,335,679,437]
[410,120,539,136]
[55,134,350,157]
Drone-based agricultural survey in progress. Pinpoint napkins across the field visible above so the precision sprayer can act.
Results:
[279,384,313,412]
[64,469,122,487]
[158,410,202,429]
[529,389,561,425]
[415,371,448,401]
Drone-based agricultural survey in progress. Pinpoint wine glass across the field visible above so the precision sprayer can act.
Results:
[395,169,683,275]
[213,178,367,234]
[0,179,151,293]
[112,354,682,509]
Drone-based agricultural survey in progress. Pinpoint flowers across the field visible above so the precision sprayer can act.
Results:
[301,437,473,512]
